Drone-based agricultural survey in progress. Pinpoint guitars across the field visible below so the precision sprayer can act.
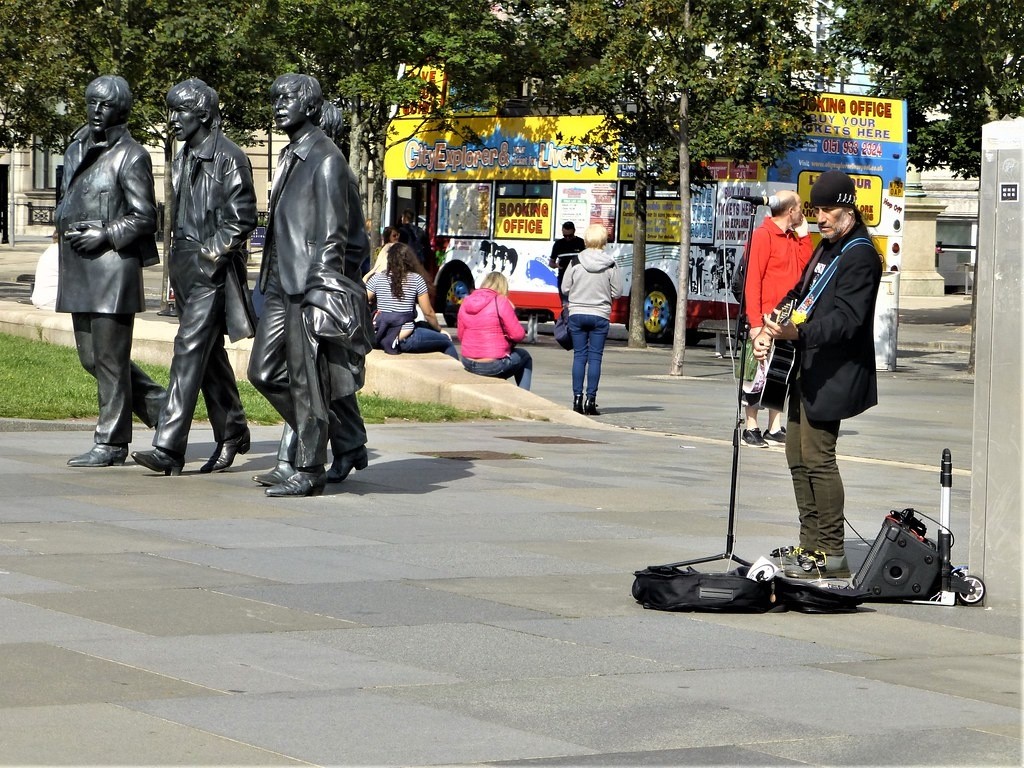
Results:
[758,299,798,413]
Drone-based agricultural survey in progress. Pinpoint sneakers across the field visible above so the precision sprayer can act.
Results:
[763,426,786,445]
[785,550,852,579]
[767,547,804,573]
[741,427,769,448]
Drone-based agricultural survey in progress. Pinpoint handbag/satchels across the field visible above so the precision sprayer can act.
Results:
[554,306,574,352]
[735,337,769,394]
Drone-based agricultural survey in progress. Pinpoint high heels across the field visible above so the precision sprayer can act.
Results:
[573,394,584,414]
[584,397,601,416]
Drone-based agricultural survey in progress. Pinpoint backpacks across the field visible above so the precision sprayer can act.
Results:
[732,253,746,304]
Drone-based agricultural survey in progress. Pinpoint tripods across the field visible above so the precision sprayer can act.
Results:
[666,204,758,567]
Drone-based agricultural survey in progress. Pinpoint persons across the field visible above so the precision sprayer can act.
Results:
[740,190,816,448]
[30,229,61,310]
[52,76,165,467]
[248,71,374,498]
[548,221,585,308]
[131,79,260,476]
[457,272,533,393]
[752,169,883,578]
[366,243,460,360]
[398,208,428,274]
[368,225,399,274]
[561,224,624,415]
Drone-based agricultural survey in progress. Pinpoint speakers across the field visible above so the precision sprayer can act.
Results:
[854,519,940,600]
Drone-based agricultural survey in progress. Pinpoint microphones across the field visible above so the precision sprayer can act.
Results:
[731,195,780,209]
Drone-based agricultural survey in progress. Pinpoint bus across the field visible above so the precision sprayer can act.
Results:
[376,57,908,342]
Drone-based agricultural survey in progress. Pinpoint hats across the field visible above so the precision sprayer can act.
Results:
[809,170,855,209]
